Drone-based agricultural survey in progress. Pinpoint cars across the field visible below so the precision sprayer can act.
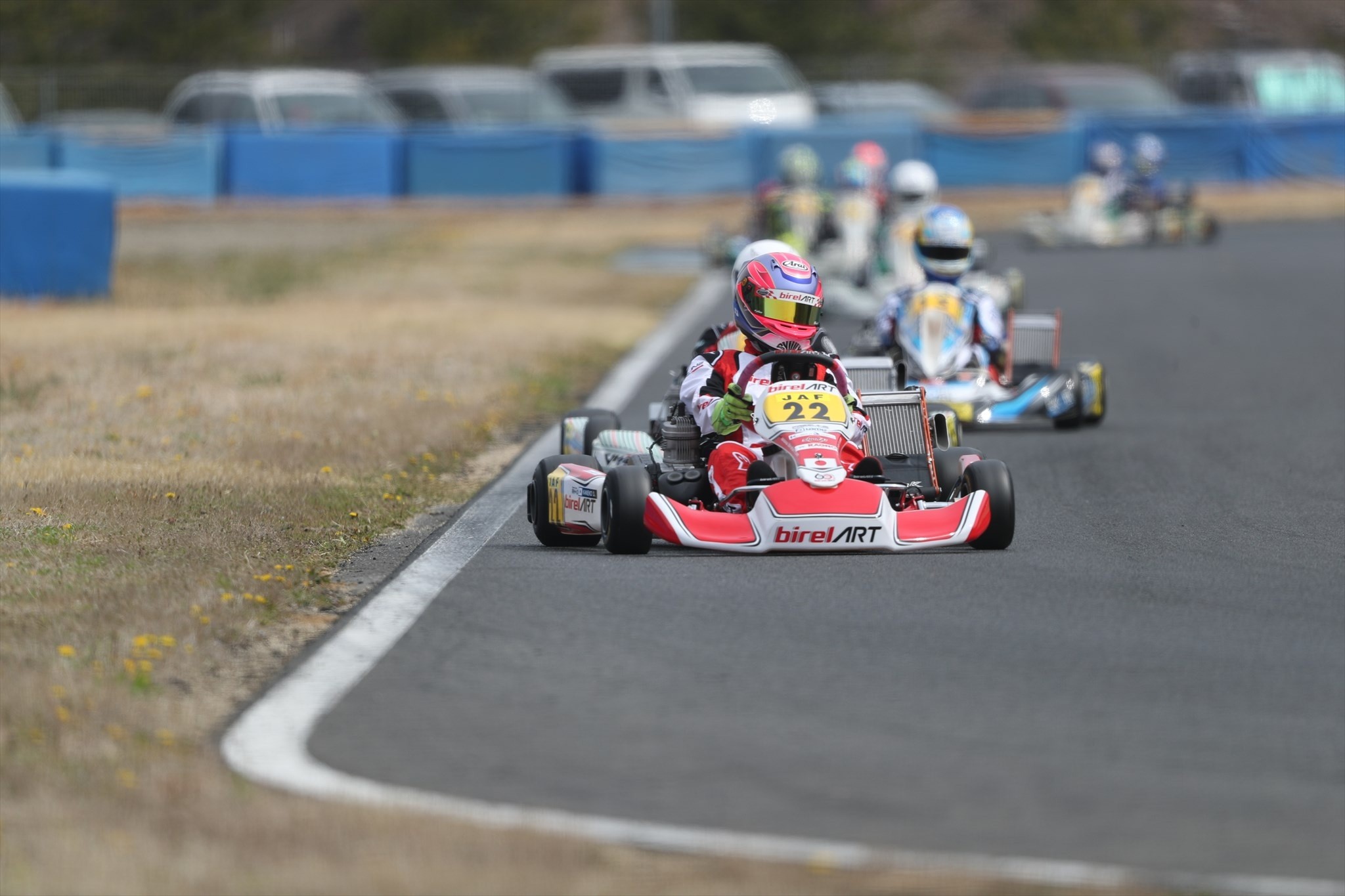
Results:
[810,76,957,131]
[959,65,1184,127]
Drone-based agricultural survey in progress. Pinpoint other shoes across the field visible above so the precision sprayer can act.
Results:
[853,456,887,485]
[744,459,778,510]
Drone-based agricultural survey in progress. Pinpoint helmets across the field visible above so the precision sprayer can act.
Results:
[835,158,868,188]
[853,140,885,171]
[733,251,824,353]
[731,239,800,289]
[915,205,973,275]
[890,160,936,202]
[780,144,818,180]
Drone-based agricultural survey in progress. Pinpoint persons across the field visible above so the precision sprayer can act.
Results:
[866,212,1010,390]
[679,241,872,515]
[753,141,942,288]
[1063,126,1193,221]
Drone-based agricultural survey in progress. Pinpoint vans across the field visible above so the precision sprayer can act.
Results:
[157,65,405,129]
[531,39,820,134]
[376,62,580,131]
[1155,43,1345,116]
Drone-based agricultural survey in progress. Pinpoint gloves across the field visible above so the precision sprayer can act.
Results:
[712,383,754,435]
[844,394,856,414]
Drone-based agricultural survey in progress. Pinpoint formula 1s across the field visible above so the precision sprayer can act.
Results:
[722,184,1108,430]
[524,351,1017,554]
[1017,170,1224,250]
